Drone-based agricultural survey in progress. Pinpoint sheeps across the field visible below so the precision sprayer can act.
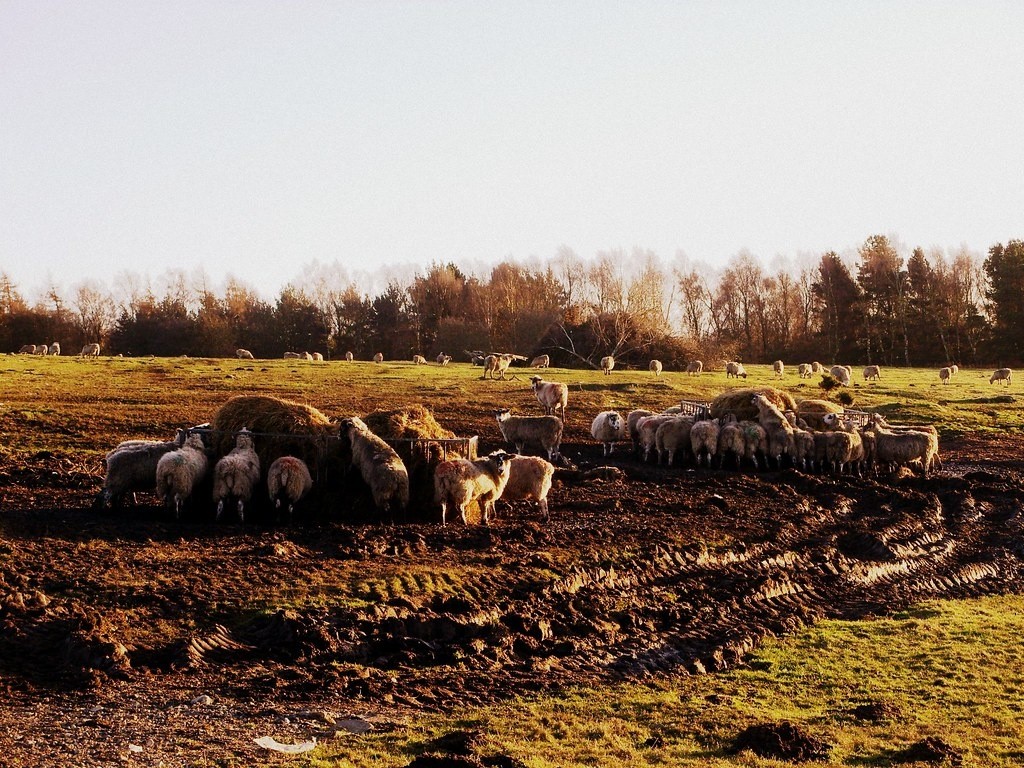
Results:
[989,368,1012,385]
[491,407,564,462]
[284,351,554,381]
[627,394,942,477]
[774,359,785,377]
[863,365,882,382]
[939,364,959,385]
[650,359,663,376]
[104,425,313,517]
[722,360,748,378]
[82,343,101,358]
[601,356,615,375]
[686,360,703,376]
[19,341,61,356]
[591,410,625,456]
[528,373,569,424]
[236,349,254,359]
[435,449,555,526]
[338,415,410,515]
[797,361,852,385]
